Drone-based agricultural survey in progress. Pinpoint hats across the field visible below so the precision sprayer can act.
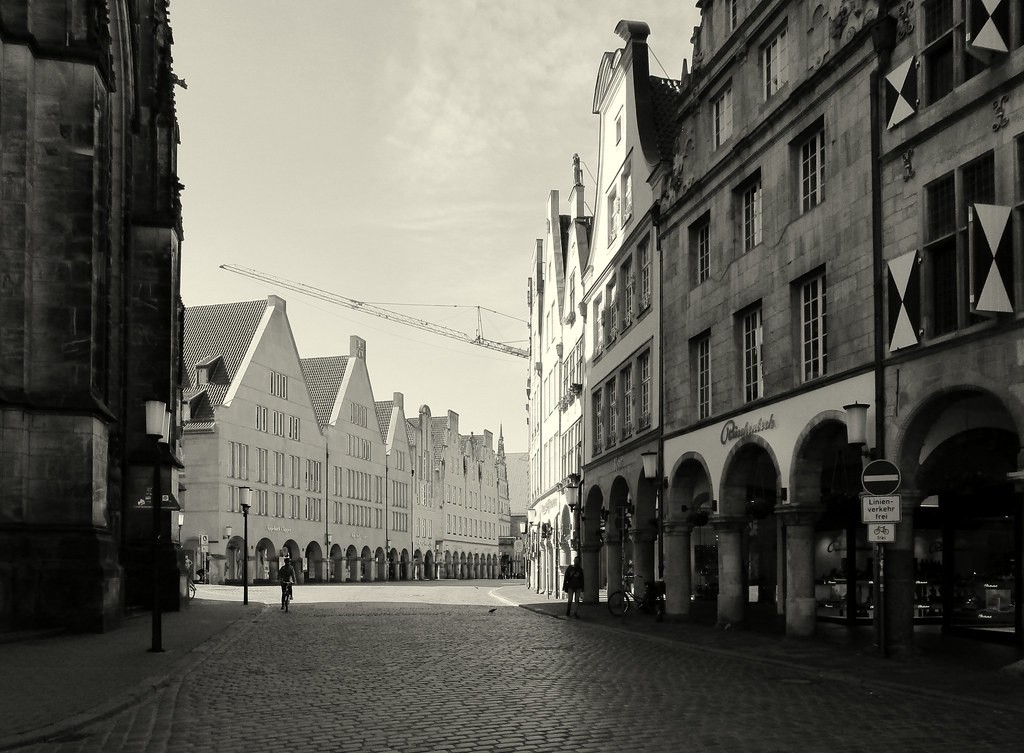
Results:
[284,558,292,562]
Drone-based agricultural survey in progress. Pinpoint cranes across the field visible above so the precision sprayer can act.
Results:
[218,262,530,360]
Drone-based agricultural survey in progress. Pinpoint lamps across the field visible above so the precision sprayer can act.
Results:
[325,534,332,545]
[566,483,585,514]
[223,525,232,539]
[641,449,668,489]
[498,551,502,557]
[843,400,877,461]
[519,521,530,537]
[527,507,540,529]
[385,539,391,550]
[434,544,439,552]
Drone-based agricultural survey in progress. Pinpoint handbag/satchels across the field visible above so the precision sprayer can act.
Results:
[565,583,568,593]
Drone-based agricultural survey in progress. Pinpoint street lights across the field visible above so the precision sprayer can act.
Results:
[238,484,253,606]
[178,513,184,545]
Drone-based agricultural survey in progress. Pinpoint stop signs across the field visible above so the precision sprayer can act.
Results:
[861,459,902,497]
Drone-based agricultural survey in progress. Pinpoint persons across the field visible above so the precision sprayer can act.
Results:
[501,564,508,574]
[277,558,296,609]
[185,555,193,569]
[564,557,584,618]
[196,567,208,583]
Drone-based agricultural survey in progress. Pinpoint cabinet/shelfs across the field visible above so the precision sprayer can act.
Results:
[814,577,943,610]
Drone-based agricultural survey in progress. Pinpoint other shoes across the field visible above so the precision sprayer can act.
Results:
[574,613,579,618]
[566,611,570,618]
[281,606,284,609]
[289,596,293,600]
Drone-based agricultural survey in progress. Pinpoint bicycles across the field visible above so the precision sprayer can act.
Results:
[605,572,665,617]
[189,584,196,601]
[280,580,294,613]
